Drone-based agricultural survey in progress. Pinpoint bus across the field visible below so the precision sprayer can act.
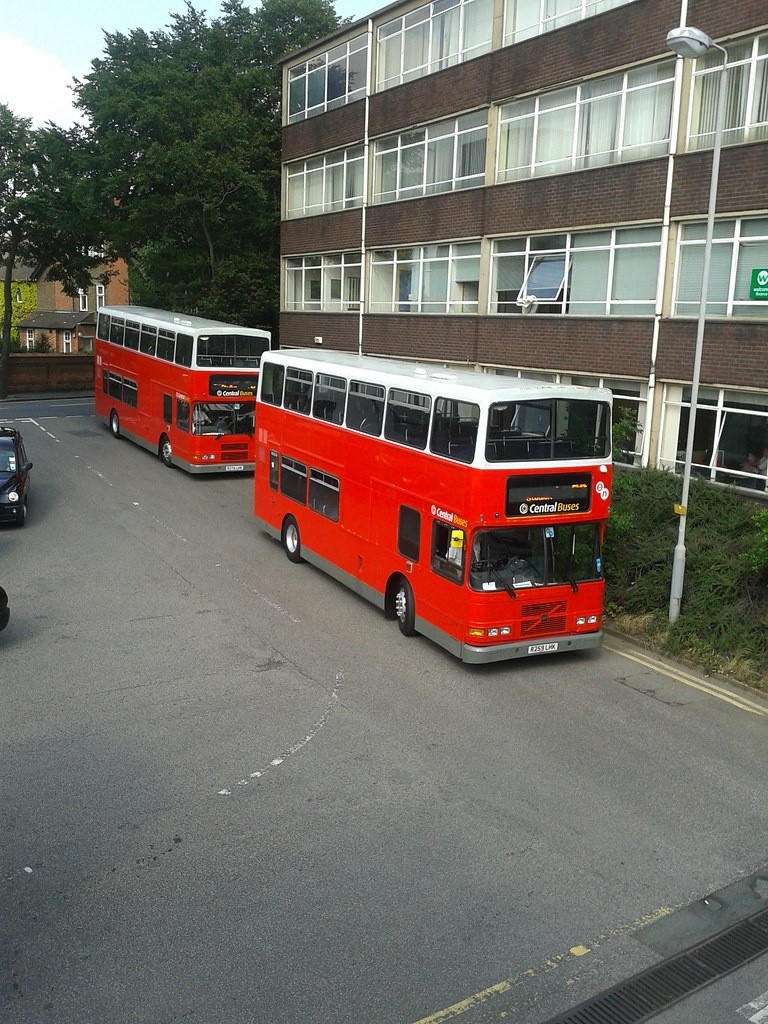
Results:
[254,347,613,664]
[95,305,272,474]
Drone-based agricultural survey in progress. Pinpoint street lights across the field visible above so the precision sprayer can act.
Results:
[665,27,727,625]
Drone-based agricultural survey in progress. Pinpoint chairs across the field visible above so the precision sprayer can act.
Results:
[392,406,410,423]
[380,405,392,423]
[404,409,424,426]
[364,400,377,418]
[296,397,310,412]
[324,405,339,421]
[338,409,358,426]
[359,416,378,433]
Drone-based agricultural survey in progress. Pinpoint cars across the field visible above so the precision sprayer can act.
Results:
[0,427,32,526]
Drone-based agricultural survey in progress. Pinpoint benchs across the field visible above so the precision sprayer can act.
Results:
[441,416,479,437]
[449,442,498,463]
[527,441,573,461]
[469,425,521,449]
[406,425,452,449]
